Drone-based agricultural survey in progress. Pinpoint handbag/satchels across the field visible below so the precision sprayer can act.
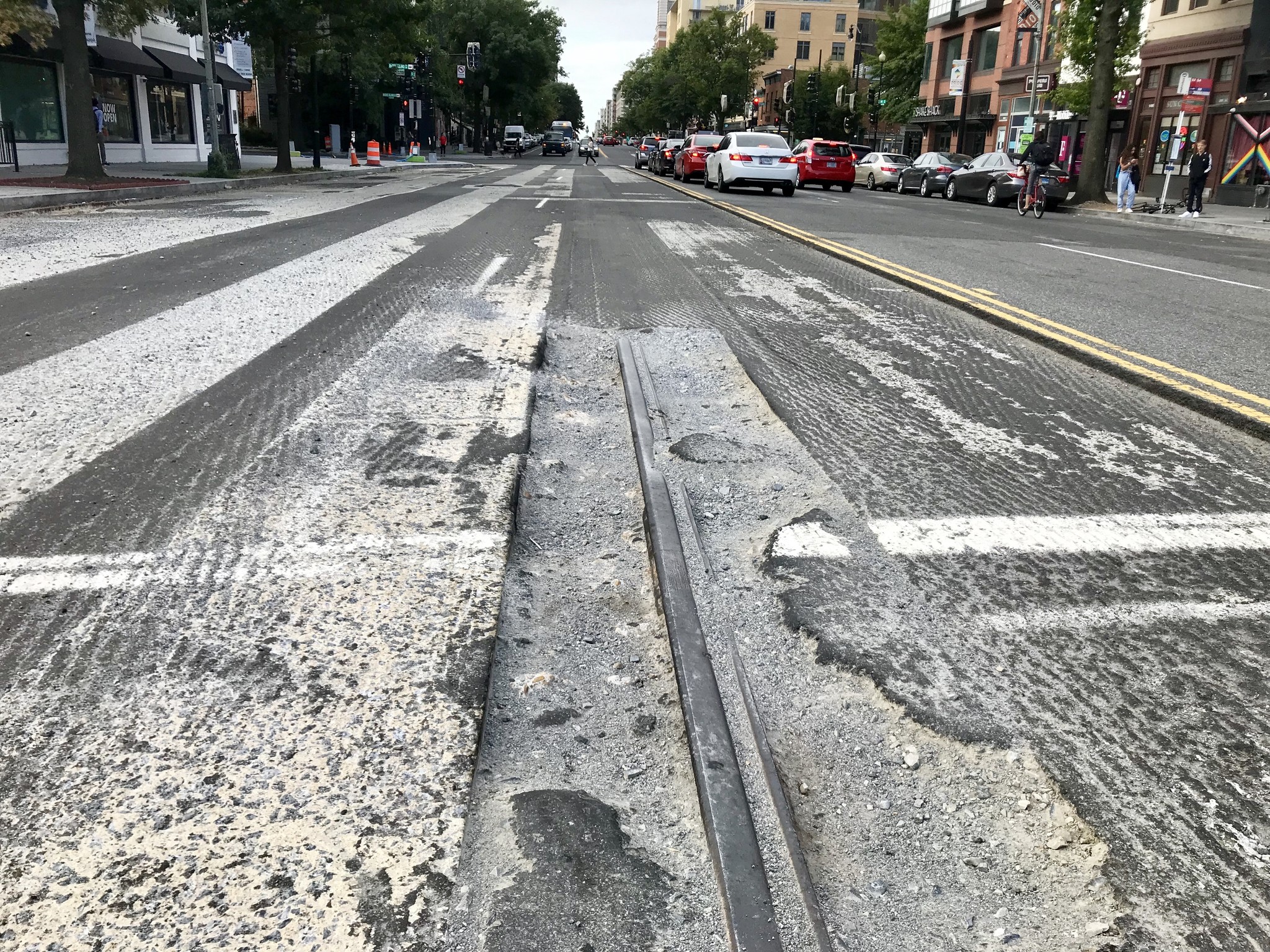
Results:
[1115,164,1121,178]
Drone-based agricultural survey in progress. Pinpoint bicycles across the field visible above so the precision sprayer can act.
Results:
[1016,164,1046,219]
[1131,188,1203,214]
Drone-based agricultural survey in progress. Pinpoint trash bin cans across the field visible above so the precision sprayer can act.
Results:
[218,133,242,170]
[484,141,492,156]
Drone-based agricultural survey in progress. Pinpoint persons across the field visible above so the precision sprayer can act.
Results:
[92,98,110,165]
[1179,139,1212,218]
[440,132,447,157]
[1017,131,1050,212]
[1116,142,1138,213]
[514,136,524,158]
[583,137,598,166]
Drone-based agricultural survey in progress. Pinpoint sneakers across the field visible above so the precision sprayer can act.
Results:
[1117,208,1122,212]
[1125,208,1132,213]
[1193,211,1199,218]
[1178,211,1191,217]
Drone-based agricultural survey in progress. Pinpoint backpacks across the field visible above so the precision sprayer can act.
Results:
[589,145,594,156]
[93,109,100,133]
[1034,139,1055,166]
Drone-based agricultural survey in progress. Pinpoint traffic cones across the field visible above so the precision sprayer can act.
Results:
[349,142,361,167]
[381,142,386,154]
[385,142,392,155]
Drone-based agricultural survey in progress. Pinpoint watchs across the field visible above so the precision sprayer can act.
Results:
[1128,161,1132,166]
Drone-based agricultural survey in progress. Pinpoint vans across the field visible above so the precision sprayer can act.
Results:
[501,126,526,153]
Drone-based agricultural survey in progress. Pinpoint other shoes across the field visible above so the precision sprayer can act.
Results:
[594,163,598,166]
[1042,185,1045,189]
[102,162,110,165]
[444,155,445,157]
[1022,208,1028,212]
[582,163,587,166]
[440,155,442,157]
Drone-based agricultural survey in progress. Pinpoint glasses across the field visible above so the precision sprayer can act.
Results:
[588,138,589,139]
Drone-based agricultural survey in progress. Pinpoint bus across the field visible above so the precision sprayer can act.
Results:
[550,121,574,141]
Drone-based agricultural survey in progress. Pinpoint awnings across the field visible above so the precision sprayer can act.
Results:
[910,114,997,138]
[142,45,206,83]
[0,18,65,61]
[197,57,252,91]
[87,35,164,78]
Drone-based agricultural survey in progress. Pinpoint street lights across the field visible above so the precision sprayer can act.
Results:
[715,48,749,132]
[871,51,886,152]
[847,22,863,143]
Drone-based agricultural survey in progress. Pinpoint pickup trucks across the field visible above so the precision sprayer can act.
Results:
[540,131,567,157]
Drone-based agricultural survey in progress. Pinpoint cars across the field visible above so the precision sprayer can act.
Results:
[897,152,974,198]
[563,137,573,152]
[944,152,1070,210]
[791,137,856,193]
[703,132,797,197]
[628,134,725,183]
[853,152,914,192]
[573,136,620,146]
[577,138,599,157]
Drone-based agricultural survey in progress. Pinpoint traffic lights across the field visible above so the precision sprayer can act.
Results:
[775,118,779,126]
[773,98,779,112]
[615,130,618,133]
[458,78,465,85]
[807,73,815,92]
[804,100,816,116]
[286,48,296,80]
[752,117,758,126]
[416,85,425,100]
[603,133,606,136]
[403,100,408,107]
[622,132,625,136]
[752,97,759,111]
[353,87,359,101]
[867,89,875,105]
[340,54,347,78]
[868,113,877,125]
[292,80,301,92]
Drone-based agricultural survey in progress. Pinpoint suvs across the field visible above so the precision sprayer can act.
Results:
[524,133,545,149]
